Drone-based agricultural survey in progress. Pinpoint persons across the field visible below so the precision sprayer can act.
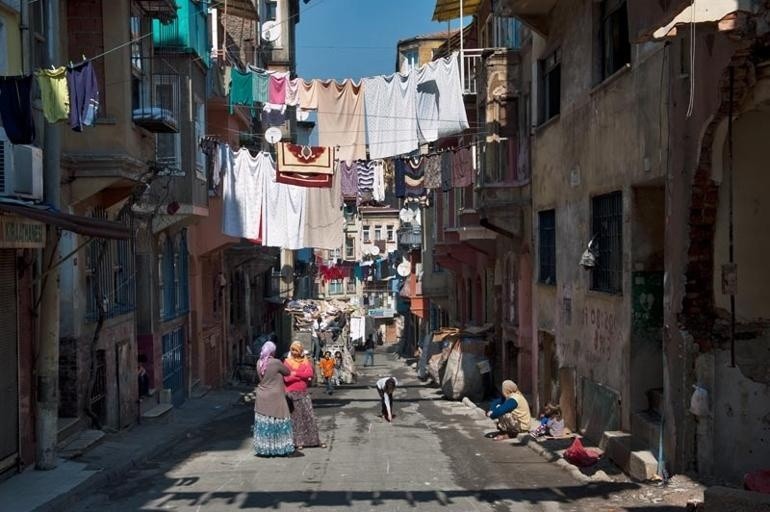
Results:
[252,340,295,458]
[362,333,375,367]
[332,347,343,387]
[320,349,337,395]
[486,378,532,442]
[376,376,398,422]
[282,341,327,451]
[529,398,565,439]
[138,352,153,401]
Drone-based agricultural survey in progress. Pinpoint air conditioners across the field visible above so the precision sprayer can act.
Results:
[0,139,43,203]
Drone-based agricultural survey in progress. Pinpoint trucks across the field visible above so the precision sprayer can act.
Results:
[291,312,356,362]
